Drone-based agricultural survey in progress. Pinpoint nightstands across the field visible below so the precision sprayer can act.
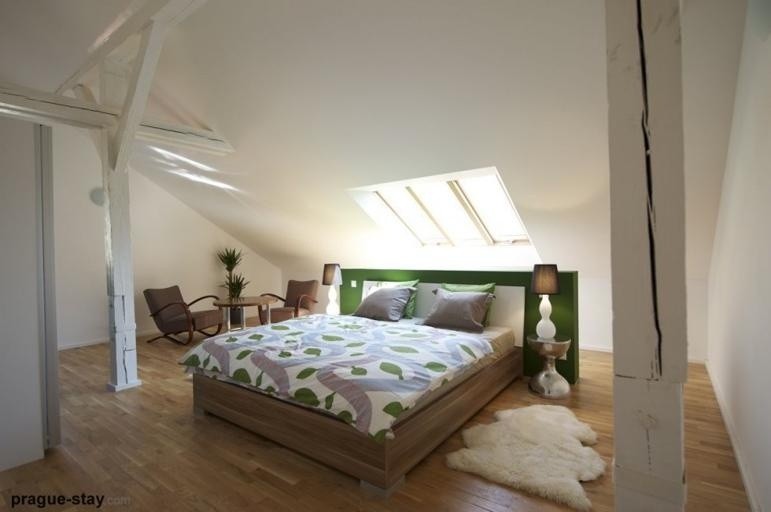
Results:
[527,335,571,400]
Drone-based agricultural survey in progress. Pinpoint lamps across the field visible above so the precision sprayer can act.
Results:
[322,264,343,315]
[530,264,560,339]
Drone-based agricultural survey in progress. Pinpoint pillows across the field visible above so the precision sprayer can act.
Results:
[349,279,419,322]
[415,282,496,334]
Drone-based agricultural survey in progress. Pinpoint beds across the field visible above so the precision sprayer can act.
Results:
[179,269,579,489]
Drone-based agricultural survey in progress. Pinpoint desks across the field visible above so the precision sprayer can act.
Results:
[213,296,278,332]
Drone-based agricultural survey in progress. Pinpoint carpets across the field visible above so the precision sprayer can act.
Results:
[447,405,607,512]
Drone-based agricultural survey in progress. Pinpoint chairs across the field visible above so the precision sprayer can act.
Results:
[258,280,319,325]
[143,285,224,345]
